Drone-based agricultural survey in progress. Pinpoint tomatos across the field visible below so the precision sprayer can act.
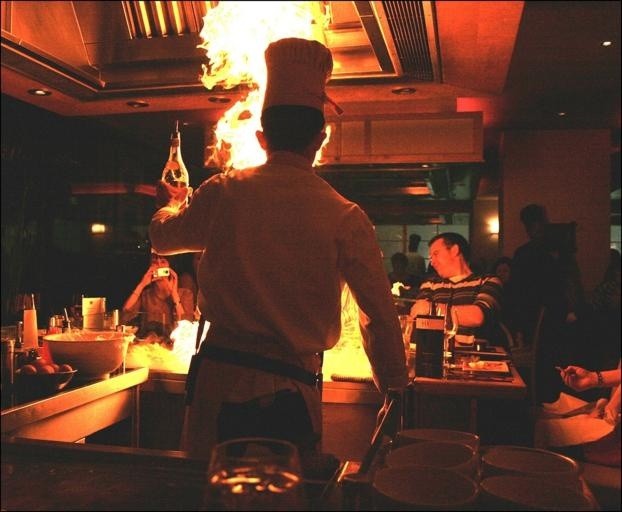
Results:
[21,358,72,375]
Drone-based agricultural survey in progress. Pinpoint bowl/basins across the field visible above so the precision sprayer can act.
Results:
[42,331,135,380]
[365,427,593,511]
[17,369,78,391]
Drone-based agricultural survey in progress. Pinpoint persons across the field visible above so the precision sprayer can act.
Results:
[584,257,621,326]
[149,95,413,480]
[386,248,419,292]
[527,356,622,452]
[123,251,193,334]
[403,231,510,351]
[488,253,532,350]
[402,233,427,273]
[513,203,589,278]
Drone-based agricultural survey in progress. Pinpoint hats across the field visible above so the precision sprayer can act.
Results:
[261,37,333,114]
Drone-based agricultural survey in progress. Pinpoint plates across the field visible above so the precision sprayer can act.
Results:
[462,359,510,375]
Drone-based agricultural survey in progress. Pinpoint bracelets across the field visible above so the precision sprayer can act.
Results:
[594,370,603,385]
[173,301,182,306]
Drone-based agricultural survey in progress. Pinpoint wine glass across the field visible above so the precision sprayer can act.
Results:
[436,306,459,359]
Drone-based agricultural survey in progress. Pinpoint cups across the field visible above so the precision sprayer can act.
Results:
[397,314,414,352]
[205,437,309,511]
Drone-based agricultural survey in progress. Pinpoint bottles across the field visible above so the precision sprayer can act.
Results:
[155,119,190,216]
[22,294,42,351]
